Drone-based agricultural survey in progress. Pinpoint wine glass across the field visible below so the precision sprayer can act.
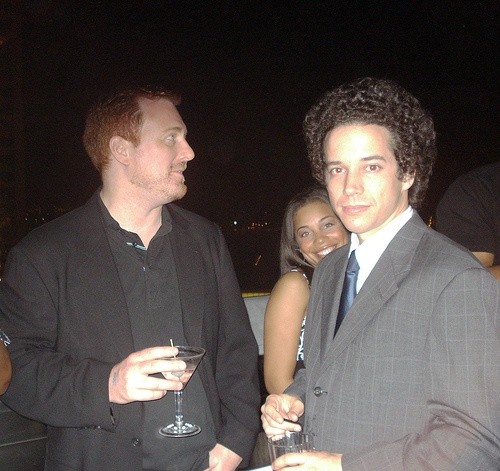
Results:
[156,345,206,438]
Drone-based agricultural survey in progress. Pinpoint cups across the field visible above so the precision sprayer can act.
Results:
[267,430,315,471]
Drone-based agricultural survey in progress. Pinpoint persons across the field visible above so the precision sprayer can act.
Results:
[0,77,263,471]
[435,162,500,283]
[264,187,350,394]
[261,78,500,471]
